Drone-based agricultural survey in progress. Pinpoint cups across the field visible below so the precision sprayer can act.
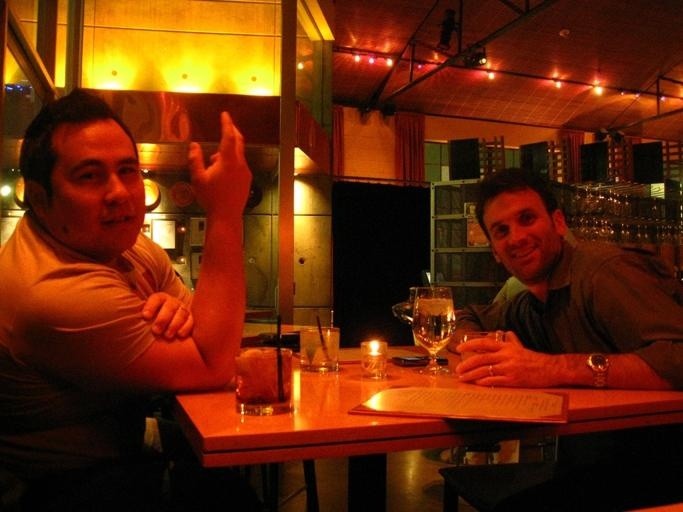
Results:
[233,326,387,414]
[460,331,503,380]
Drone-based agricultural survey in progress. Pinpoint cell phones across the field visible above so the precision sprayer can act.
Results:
[392,354,449,366]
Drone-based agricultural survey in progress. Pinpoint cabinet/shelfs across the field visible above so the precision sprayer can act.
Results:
[429,180,683,290]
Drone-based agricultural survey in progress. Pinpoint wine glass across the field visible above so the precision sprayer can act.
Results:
[412,286,457,376]
[573,181,682,243]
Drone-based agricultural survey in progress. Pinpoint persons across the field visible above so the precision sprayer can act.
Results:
[0,87,250,511]
[449,165,683,512]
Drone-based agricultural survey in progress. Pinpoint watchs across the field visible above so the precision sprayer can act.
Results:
[587,351,610,388]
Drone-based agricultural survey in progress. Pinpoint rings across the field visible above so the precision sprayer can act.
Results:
[488,365,493,376]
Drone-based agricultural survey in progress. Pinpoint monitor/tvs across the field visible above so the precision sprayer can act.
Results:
[632,141,664,183]
[580,141,608,183]
[519,141,550,180]
[448,138,481,180]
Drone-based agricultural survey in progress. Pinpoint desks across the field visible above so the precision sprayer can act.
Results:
[172,345,681,511]
